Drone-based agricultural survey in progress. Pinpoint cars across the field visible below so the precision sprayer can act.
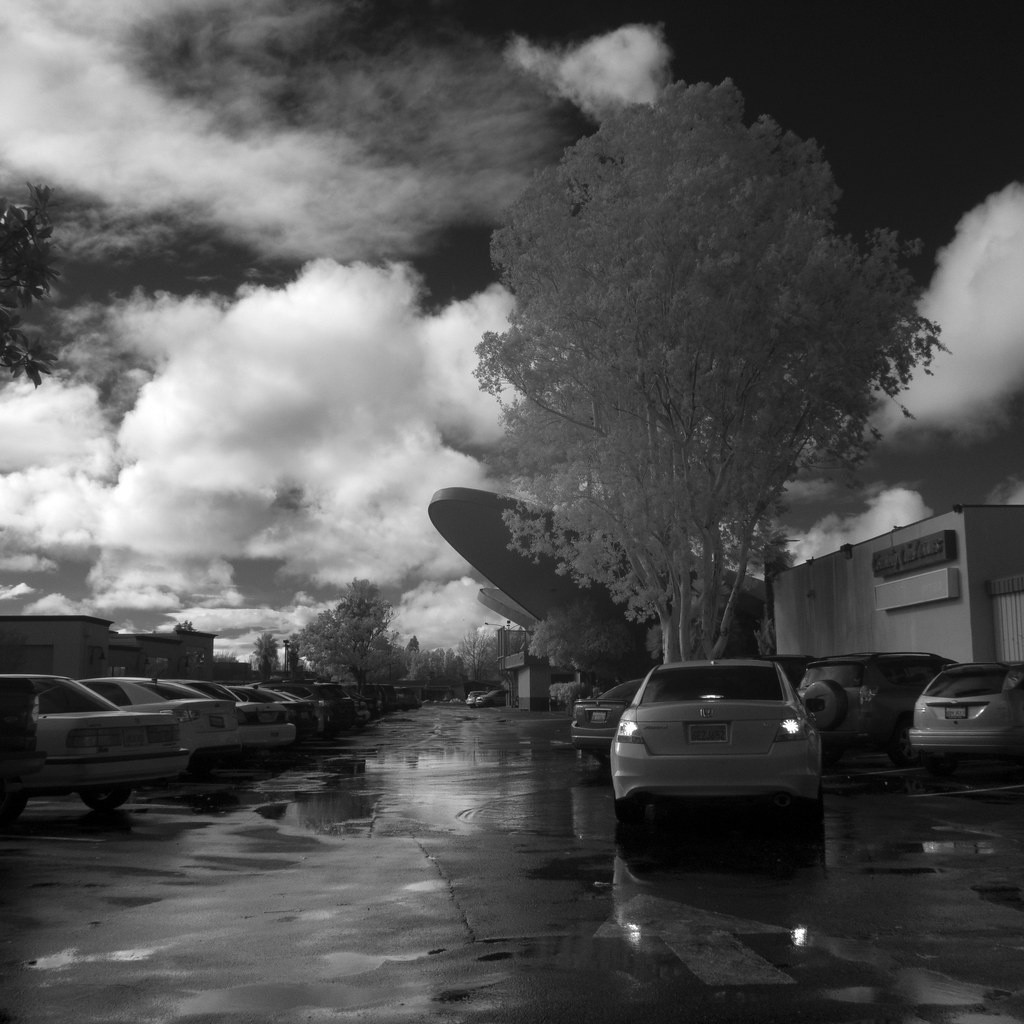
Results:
[571,678,645,766]
[34,676,242,786]
[466,690,490,708]
[212,675,423,768]
[475,689,509,708]
[609,657,823,825]
[748,653,817,692]
[0,673,190,819]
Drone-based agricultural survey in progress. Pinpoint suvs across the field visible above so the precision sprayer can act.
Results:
[908,660,1024,783]
[798,651,962,768]
